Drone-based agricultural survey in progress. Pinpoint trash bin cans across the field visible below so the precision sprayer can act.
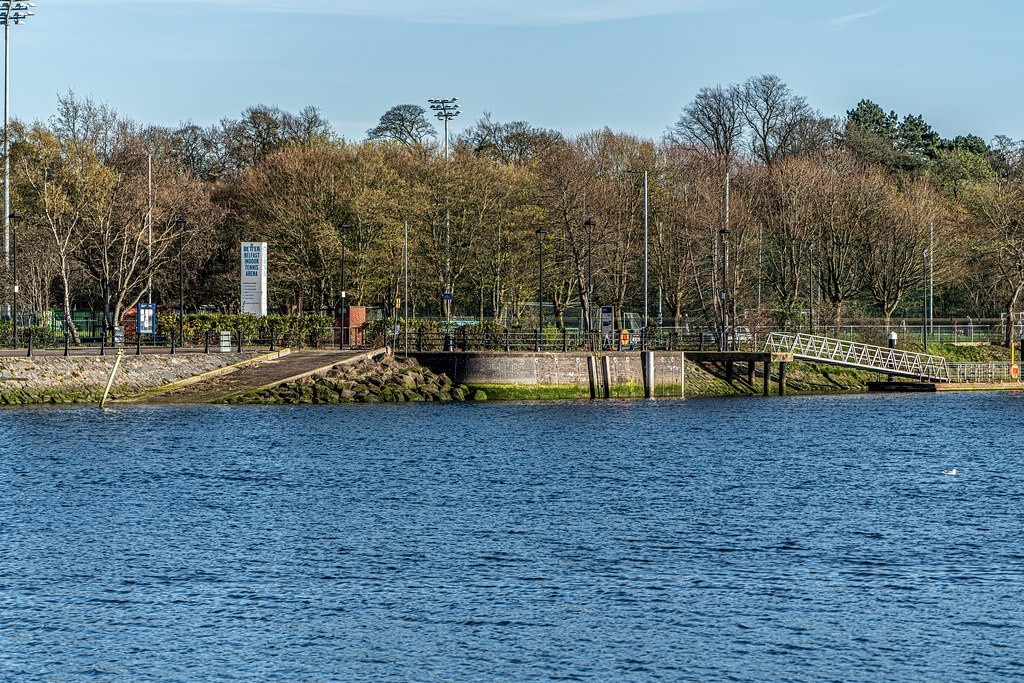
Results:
[443,334,454,352]
[111,326,125,347]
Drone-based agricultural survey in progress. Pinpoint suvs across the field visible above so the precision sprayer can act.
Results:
[703,326,754,345]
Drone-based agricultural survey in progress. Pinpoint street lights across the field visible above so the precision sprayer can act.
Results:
[582,218,596,350]
[7,211,20,351]
[428,97,463,316]
[808,243,816,345]
[0,0,37,326]
[337,224,351,349]
[623,169,649,329]
[719,227,731,351]
[534,227,547,352]
[176,216,187,348]
[921,248,929,352]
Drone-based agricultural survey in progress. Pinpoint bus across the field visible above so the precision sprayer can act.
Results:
[500,302,604,330]
